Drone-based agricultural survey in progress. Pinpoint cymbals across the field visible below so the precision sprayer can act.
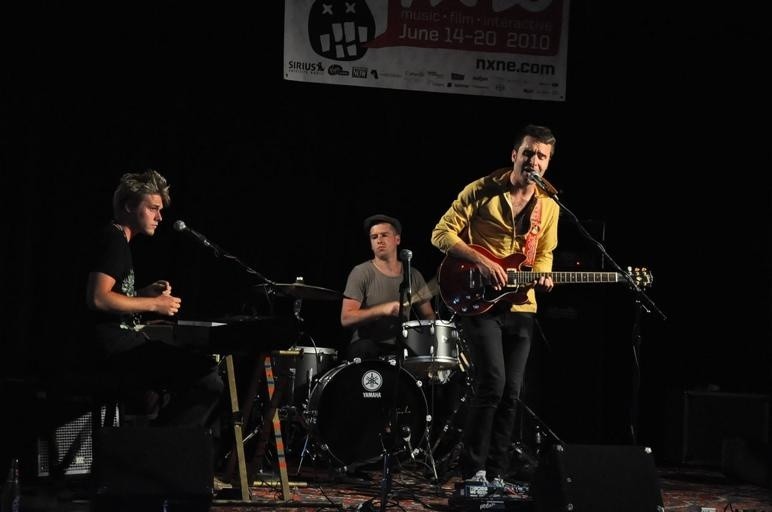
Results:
[250,282,341,302]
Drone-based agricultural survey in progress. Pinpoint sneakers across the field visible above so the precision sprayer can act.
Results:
[213,477,232,491]
[460,469,505,497]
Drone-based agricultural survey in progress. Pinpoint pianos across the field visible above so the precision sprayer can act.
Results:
[145,307,278,355]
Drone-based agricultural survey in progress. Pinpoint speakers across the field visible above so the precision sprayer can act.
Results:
[89,425,216,509]
[531,442,664,511]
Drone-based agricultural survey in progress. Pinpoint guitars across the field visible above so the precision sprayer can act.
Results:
[437,244,659,317]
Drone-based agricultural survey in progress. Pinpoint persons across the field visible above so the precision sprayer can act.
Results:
[430,127,561,497]
[90,169,226,423]
[340,214,438,357]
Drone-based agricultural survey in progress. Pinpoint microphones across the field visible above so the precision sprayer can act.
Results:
[525,167,557,200]
[400,245,415,298]
[170,216,212,248]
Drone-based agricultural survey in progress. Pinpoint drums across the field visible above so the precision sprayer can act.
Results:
[307,354,429,480]
[400,319,460,373]
[274,346,338,392]
